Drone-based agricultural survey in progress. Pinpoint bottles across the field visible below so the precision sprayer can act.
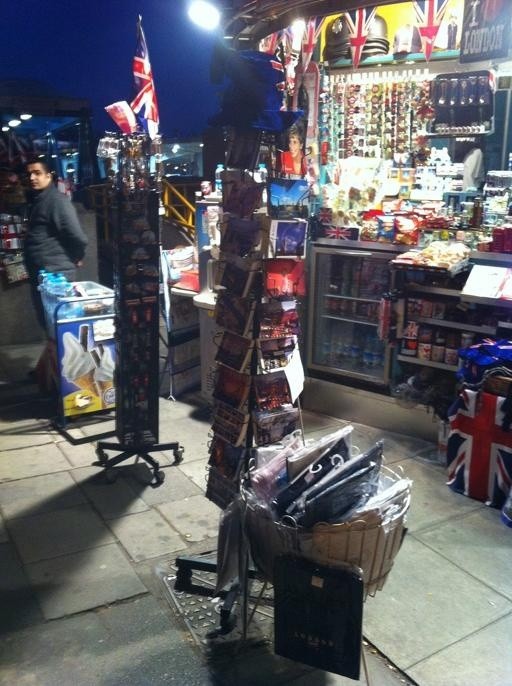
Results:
[443,195,512,253]
[255,161,268,181]
[214,163,226,196]
[37,268,82,319]
[318,252,386,377]
[94,130,165,159]
[401,266,478,367]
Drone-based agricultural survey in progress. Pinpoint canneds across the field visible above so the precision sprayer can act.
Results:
[491,227,503,252]
[401,297,475,365]
[503,227,512,254]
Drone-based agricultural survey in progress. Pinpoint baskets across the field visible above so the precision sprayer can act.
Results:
[237,445,412,602]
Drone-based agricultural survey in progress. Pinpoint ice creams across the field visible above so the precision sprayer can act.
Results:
[60,325,97,398]
[91,344,115,409]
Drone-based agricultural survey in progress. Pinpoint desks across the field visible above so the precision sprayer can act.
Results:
[170,286,222,405]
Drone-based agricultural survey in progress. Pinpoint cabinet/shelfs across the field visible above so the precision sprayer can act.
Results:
[395,244,512,411]
[55,279,117,446]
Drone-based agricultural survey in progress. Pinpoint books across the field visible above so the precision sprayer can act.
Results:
[206,175,307,510]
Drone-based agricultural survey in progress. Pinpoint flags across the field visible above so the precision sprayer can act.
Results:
[127,19,159,140]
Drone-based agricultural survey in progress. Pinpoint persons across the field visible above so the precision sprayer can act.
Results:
[20,156,88,333]
[278,125,307,175]
[200,180,212,195]
[455,143,485,191]
